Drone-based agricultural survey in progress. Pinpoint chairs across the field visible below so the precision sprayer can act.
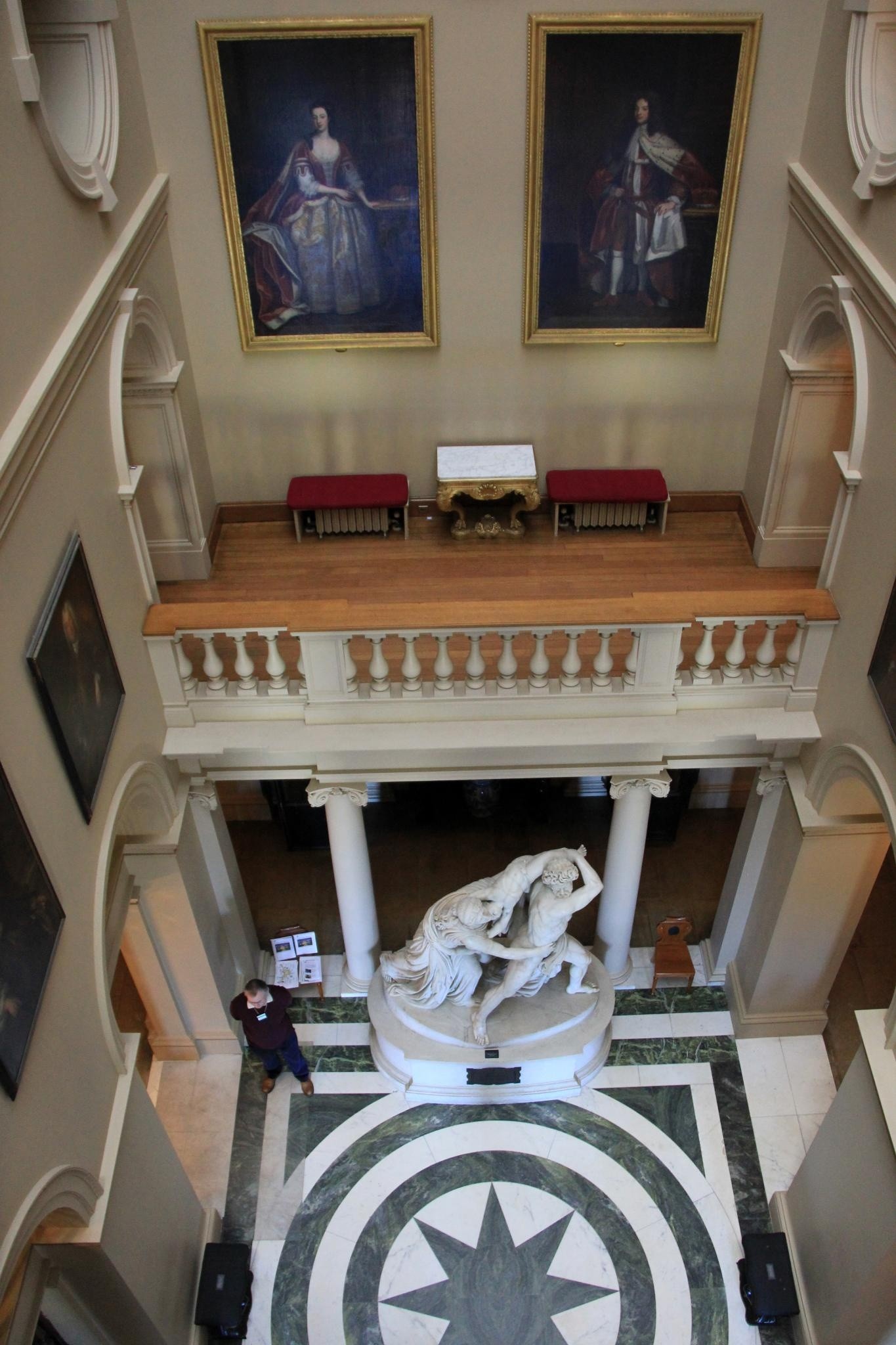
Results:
[273,924,325,1003]
[651,917,696,995]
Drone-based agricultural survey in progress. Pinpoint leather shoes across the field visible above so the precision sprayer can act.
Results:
[301,1080,313,1097]
[262,1077,275,1094]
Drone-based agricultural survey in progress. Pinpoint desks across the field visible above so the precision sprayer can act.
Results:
[193,1242,253,1339]
[436,445,541,541]
[736,1232,800,1326]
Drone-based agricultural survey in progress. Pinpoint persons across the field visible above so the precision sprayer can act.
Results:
[380,845,604,1046]
[230,978,314,1097]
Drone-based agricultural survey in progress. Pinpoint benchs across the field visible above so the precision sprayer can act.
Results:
[285,472,411,544]
[544,469,671,537]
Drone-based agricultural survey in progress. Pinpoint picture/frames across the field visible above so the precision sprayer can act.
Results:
[197,15,442,351]
[25,528,126,826]
[521,11,766,348]
[0,761,66,1101]
[866,575,896,744]
